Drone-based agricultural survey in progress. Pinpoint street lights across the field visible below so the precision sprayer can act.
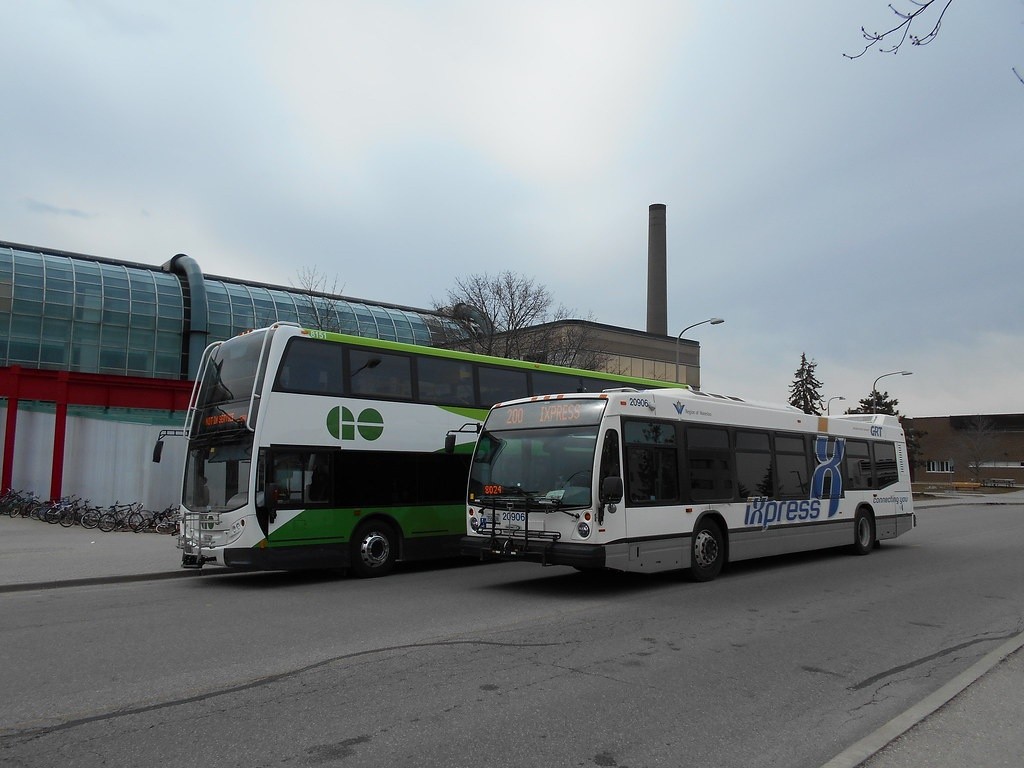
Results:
[827,397,846,416]
[674,318,726,383]
[873,371,914,415]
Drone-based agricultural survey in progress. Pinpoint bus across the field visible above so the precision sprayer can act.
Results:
[151,319,697,581]
[442,382,919,584]
